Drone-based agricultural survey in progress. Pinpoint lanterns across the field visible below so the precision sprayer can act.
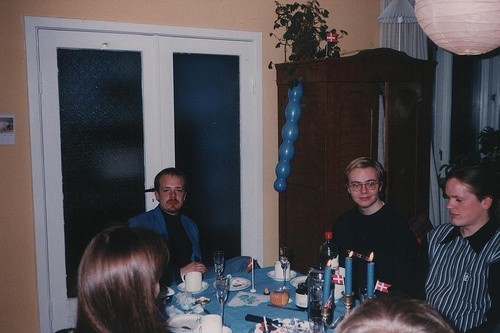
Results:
[414,0,500,55]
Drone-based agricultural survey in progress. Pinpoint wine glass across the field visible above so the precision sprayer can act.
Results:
[278,247,291,292]
[215,278,231,327]
[212,251,224,297]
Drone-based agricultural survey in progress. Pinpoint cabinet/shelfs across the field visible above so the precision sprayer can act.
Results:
[276,48,439,275]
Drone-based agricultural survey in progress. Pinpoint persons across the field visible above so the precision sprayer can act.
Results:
[56,226,174,333]
[424,164,500,333]
[131,167,206,286]
[331,157,412,292]
[332,292,455,333]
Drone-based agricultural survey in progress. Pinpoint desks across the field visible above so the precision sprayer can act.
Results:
[167,265,361,333]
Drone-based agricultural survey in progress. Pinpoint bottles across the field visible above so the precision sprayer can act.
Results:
[318,231,339,268]
[294,263,334,325]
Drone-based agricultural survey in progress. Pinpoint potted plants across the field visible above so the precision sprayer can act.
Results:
[268,0,348,90]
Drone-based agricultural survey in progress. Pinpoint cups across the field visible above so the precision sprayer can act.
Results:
[198,314,222,333]
[274,261,290,280]
[182,271,202,290]
[309,317,324,333]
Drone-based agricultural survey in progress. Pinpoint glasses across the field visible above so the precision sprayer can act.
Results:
[349,180,381,192]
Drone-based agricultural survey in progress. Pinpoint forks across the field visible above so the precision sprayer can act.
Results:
[166,326,192,331]
[325,315,344,329]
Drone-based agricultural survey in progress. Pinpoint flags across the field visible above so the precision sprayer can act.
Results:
[247,258,252,271]
[375,281,392,292]
[332,275,343,283]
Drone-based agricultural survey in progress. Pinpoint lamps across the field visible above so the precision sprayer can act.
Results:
[378,0,418,51]
[415,0,500,56]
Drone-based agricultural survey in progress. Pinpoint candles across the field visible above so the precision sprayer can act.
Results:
[323,260,333,306]
[344,250,354,295]
[367,252,375,297]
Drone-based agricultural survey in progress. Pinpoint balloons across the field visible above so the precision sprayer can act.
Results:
[274,84,302,191]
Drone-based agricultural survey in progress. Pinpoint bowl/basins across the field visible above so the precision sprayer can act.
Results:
[333,283,346,304]
[165,286,175,303]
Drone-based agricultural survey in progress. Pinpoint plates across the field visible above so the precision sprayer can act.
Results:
[166,315,204,333]
[290,276,308,287]
[178,280,209,294]
[194,326,232,333]
[213,278,250,292]
[267,270,297,282]
